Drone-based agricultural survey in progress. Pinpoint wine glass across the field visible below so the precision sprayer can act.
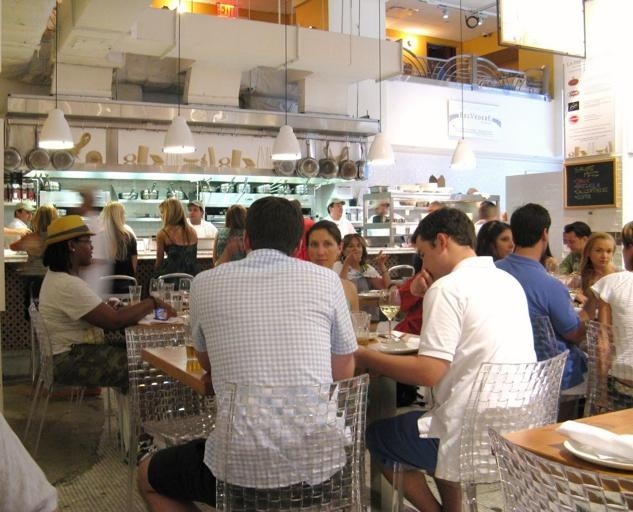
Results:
[377,287,402,344]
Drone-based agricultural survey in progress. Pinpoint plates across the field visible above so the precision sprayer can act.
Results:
[560,433,633,471]
[378,336,420,353]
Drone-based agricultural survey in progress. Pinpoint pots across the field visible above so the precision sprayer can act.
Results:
[4,121,77,170]
[40,172,311,200]
[274,133,371,180]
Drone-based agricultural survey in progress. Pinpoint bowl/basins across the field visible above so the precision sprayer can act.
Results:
[368,183,453,206]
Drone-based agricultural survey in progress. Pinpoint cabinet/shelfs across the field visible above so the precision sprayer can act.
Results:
[118,179,191,222]
[362,185,501,248]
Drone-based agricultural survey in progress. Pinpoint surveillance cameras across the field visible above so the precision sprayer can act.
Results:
[481,32,488,38]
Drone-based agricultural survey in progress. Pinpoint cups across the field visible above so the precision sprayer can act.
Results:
[546,262,560,281]
[349,311,371,346]
[185,333,205,374]
[127,277,195,360]
[566,271,582,300]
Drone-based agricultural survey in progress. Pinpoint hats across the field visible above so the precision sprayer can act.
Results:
[326,197,347,207]
[13,203,36,216]
[187,199,204,211]
[44,213,98,244]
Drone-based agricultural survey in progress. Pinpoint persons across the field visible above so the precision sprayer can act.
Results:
[5,202,57,321]
[76,188,247,306]
[474,199,633,399]
[289,198,445,407]
[353,204,545,512]
[36,212,161,466]
[0,410,63,512]
[135,194,359,512]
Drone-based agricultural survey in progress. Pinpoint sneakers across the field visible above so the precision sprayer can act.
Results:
[124,430,156,462]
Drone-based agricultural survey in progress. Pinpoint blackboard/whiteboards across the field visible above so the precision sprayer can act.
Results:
[563,158,616,210]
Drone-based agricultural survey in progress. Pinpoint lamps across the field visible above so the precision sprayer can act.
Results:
[270,0,302,161]
[439,5,449,20]
[448,1,482,174]
[367,1,395,166]
[39,0,74,151]
[163,1,198,153]
